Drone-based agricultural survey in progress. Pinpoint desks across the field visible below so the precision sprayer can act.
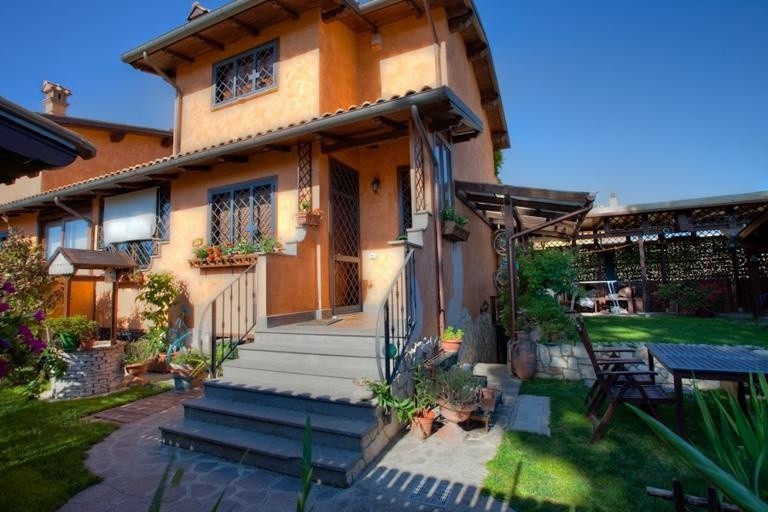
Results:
[645,341,768,441]
[593,295,635,314]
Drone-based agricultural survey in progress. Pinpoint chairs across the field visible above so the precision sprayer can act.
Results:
[574,321,675,445]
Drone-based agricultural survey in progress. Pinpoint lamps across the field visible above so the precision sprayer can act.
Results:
[371,178,380,192]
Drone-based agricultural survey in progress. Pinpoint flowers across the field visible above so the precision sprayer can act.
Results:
[189,240,282,260]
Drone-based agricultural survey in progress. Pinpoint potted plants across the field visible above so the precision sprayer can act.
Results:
[43,314,101,352]
[408,325,487,440]
[443,205,472,242]
[649,280,730,318]
[499,246,580,383]
[296,201,322,227]
[118,271,204,393]
